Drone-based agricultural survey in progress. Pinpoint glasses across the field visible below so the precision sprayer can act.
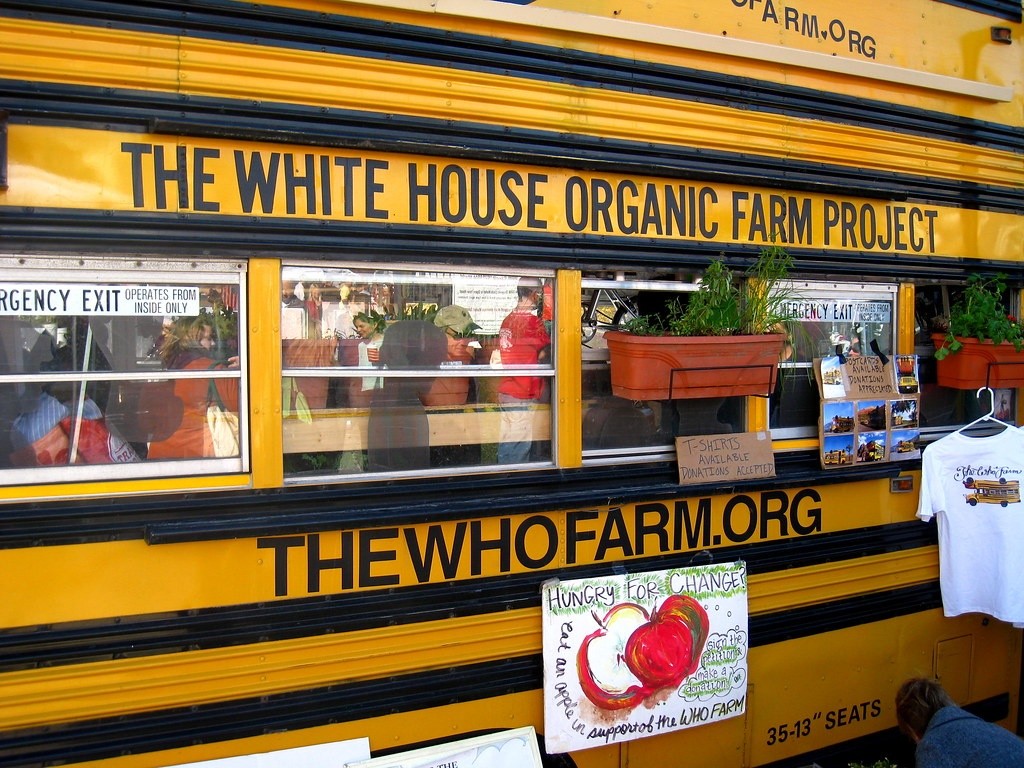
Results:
[446,331,474,339]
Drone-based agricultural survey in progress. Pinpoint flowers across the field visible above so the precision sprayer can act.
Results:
[1007,314,1017,323]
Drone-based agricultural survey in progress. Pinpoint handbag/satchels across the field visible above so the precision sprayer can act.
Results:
[206,359,241,457]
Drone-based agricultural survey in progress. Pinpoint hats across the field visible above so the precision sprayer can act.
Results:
[433,305,482,333]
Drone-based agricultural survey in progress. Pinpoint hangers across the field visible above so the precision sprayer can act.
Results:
[957,387,1010,432]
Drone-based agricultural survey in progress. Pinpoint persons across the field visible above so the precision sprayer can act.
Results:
[895,679,1024,768]
[284,282,396,321]
[354,276,554,473]
[147,317,242,458]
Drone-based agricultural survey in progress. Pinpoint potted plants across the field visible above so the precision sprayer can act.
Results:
[932,273,1024,389]
[602,230,831,401]
